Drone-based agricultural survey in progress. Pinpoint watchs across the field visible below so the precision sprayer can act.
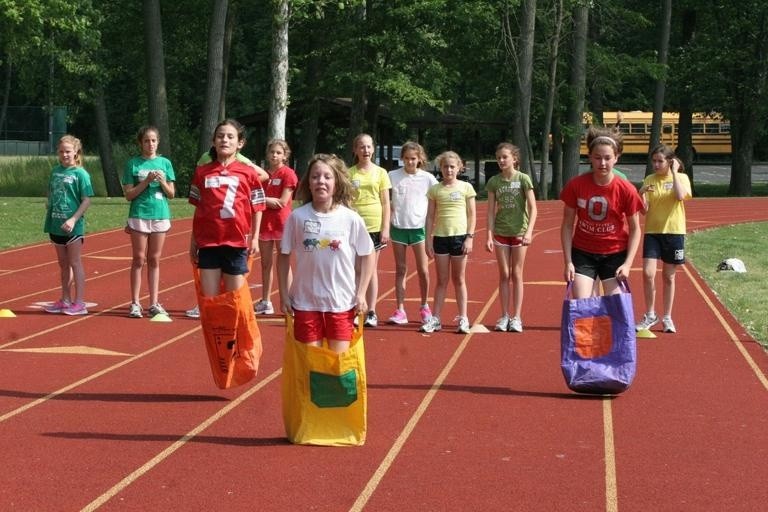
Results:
[466,234,474,238]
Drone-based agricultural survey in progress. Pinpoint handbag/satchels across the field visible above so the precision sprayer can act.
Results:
[560,275,637,396]
[190,253,263,390]
[280,308,369,447]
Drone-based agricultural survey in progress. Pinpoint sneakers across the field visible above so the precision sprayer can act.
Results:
[662,311,677,335]
[494,312,523,333]
[148,303,169,317]
[128,303,144,318]
[419,306,443,334]
[42,298,68,314]
[452,313,469,336]
[185,304,200,318]
[635,312,661,333]
[254,300,275,315]
[364,311,378,328]
[61,303,89,315]
[386,309,409,325]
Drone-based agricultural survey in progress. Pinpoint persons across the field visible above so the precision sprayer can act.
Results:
[383,140,440,326]
[188,118,267,300]
[185,120,270,319]
[42,134,95,316]
[558,124,692,333]
[275,150,376,355]
[485,140,536,333]
[122,125,177,318]
[340,131,393,329]
[418,150,477,334]
[251,138,299,314]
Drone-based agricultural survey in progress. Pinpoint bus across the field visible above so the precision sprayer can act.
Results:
[550,107,733,168]
